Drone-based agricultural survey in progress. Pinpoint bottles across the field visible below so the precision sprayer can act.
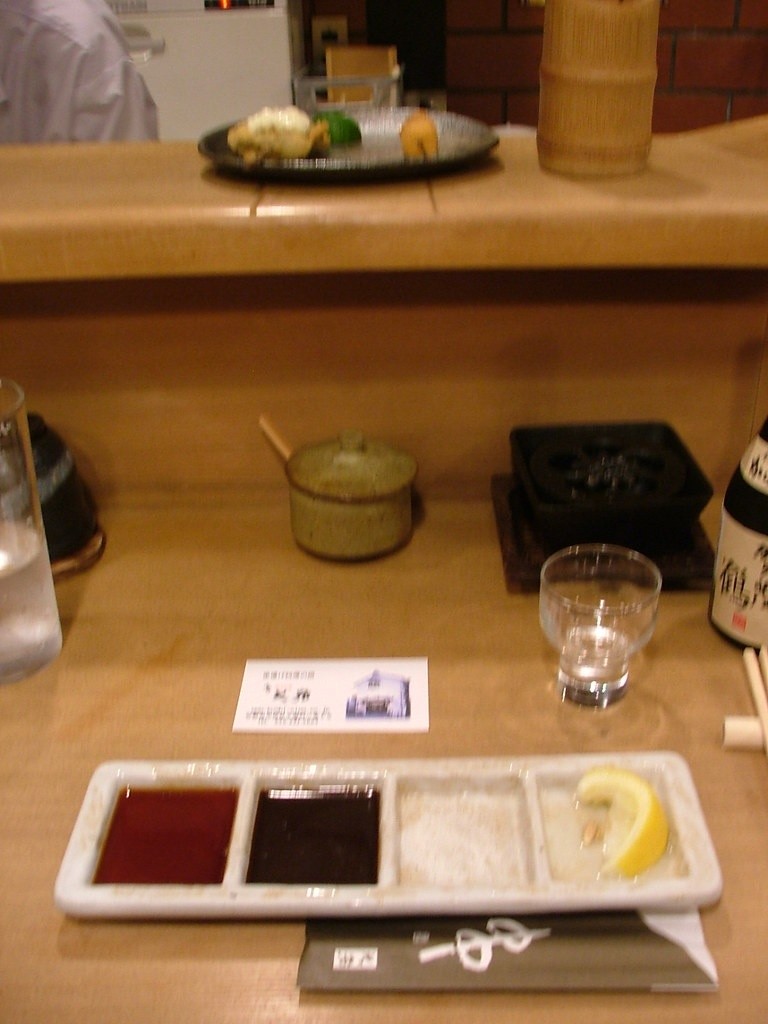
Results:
[707,417,768,657]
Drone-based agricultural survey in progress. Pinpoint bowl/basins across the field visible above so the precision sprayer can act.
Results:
[508,419,715,553]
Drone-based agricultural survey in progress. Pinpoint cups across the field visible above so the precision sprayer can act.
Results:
[537,543,662,708]
[0,378,62,684]
[284,428,417,560]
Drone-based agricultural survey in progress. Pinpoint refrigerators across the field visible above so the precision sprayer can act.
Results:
[104,1,303,139]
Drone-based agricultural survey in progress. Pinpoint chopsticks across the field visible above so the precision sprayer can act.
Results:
[743,646,768,755]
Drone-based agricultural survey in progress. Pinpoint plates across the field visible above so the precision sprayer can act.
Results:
[198,106,500,186]
[55,750,723,919]
[491,472,718,591]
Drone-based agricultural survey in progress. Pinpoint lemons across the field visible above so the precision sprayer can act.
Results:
[577,769,668,877]
[313,111,363,145]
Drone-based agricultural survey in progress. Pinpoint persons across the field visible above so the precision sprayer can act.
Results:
[0,0,160,144]
